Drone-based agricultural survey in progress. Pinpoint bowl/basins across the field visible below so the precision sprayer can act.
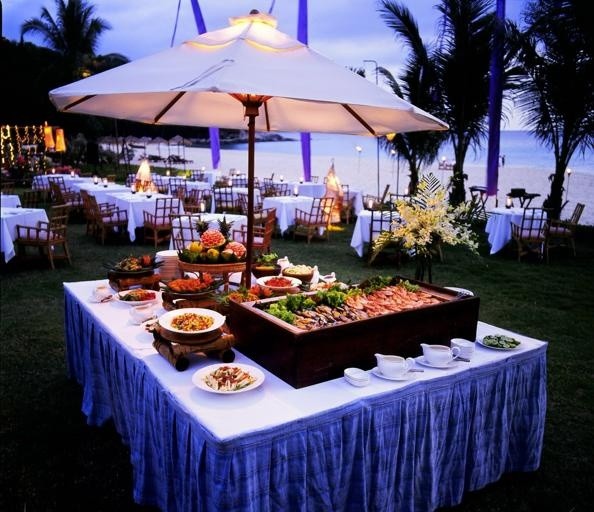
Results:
[251,264,282,279]
[281,267,313,283]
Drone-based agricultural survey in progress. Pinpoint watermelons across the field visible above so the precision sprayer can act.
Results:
[224,241,247,258]
[201,230,226,251]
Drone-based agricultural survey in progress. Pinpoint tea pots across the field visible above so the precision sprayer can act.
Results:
[420,343,461,365]
[91,284,108,297]
[371,353,415,377]
[127,302,157,324]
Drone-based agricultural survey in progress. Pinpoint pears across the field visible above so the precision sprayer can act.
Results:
[207,247,219,260]
[190,240,202,253]
[221,249,233,259]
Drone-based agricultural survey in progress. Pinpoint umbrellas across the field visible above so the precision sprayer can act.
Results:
[48,8,450,291]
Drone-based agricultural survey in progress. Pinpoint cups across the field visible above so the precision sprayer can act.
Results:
[225,169,374,209]
[69,170,186,199]
[506,198,512,209]
[200,203,205,213]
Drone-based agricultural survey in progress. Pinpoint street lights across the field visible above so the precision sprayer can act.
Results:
[354,145,363,173]
[565,168,572,200]
[390,150,396,178]
[174,135,183,161]
[441,157,447,181]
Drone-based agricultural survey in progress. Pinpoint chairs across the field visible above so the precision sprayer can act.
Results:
[288,176,587,268]
[0,145,288,278]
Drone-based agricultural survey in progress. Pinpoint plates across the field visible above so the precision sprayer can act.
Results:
[477,335,524,350]
[342,368,370,386]
[412,356,455,370]
[114,288,161,305]
[449,337,476,360]
[110,270,150,275]
[371,366,410,381]
[156,306,224,336]
[155,249,184,280]
[4,208,25,214]
[165,291,213,300]
[254,275,302,289]
[189,362,267,396]
